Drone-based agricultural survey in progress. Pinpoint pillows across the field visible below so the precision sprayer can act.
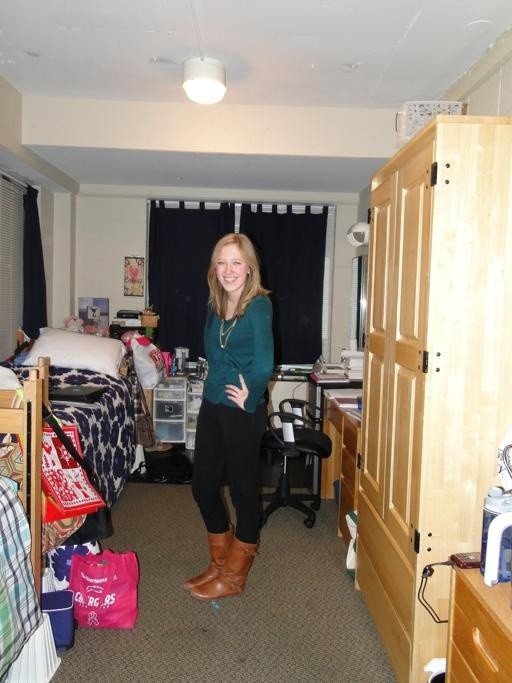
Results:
[20,326,129,380]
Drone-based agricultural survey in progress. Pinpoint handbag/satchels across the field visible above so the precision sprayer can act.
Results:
[127,454,193,484]
[32,426,139,629]
[127,329,167,389]
[134,416,155,448]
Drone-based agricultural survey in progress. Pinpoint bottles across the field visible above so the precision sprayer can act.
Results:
[195,360,207,379]
[170,360,177,377]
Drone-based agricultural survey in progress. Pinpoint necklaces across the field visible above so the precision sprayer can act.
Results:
[219,313,237,349]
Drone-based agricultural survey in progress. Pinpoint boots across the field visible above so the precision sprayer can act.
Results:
[183,524,260,601]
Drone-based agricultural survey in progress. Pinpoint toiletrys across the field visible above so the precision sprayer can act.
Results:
[196,358,208,380]
[181,352,187,375]
[169,355,178,376]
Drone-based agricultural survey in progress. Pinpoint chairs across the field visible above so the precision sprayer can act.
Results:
[257,380,333,530]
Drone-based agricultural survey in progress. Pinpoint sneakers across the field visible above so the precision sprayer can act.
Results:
[144,441,173,452]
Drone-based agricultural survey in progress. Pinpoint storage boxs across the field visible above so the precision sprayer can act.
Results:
[133,370,205,469]
[389,99,464,155]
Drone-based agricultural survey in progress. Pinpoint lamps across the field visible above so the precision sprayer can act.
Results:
[178,54,228,107]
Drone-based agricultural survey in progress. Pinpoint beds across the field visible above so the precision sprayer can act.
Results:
[0,365,43,682]
[1,333,146,563]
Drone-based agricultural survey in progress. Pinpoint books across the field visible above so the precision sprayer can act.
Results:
[311,349,365,423]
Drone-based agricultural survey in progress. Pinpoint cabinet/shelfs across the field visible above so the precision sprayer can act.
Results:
[351,107,512,683]
[438,557,512,683]
[320,389,362,552]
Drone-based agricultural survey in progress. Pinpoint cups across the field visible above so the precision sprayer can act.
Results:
[479,495,512,587]
[174,346,186,372]
[356,395,362,410]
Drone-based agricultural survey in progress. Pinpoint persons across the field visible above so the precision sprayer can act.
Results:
[186,233,271,600]
